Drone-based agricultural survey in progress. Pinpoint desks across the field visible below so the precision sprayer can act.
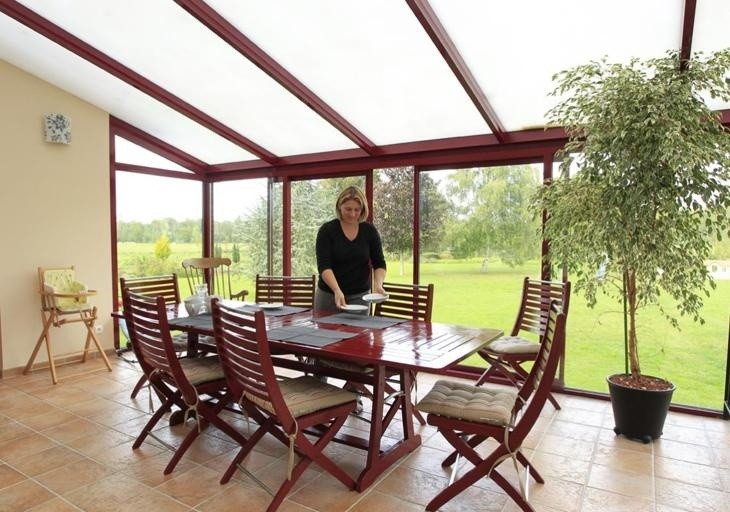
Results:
[110,305,504,493]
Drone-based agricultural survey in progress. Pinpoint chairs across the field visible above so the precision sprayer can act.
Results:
[415,300,567,512]
[475,277,573,411]
[344,282,434,437]
[255,274,315,306]
[23,264,113,385]
[119,273,181,413]
[123,286,249,476]
[209,297,360,512]
[181,256,250,305]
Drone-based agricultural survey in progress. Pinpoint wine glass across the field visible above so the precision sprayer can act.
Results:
[197,284,208,309]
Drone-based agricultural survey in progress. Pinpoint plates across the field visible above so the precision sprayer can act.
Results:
[259,304,283,310]
[340,304,367,313]
[361,293,387,304]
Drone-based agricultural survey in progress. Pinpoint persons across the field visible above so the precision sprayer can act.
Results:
[313,187,388,415]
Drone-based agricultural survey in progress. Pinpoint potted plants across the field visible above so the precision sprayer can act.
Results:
[526,44,730,444]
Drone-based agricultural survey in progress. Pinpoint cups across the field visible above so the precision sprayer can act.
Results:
[185,297,201,315]
